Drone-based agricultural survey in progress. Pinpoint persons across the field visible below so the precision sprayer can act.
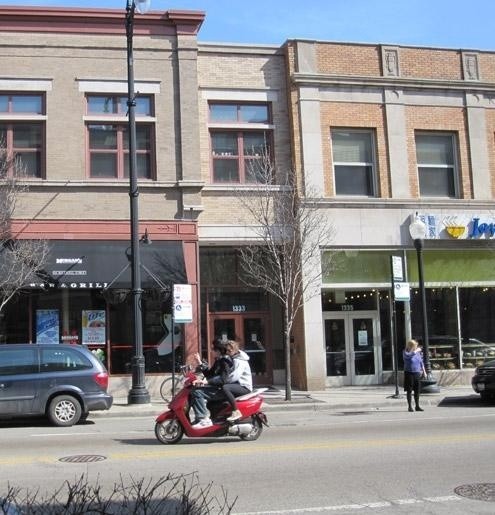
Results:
[403,340,427,411]
[191,340,252,430]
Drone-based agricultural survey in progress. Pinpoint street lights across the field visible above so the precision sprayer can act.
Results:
[124,0,150,404]
[408,211,440,394]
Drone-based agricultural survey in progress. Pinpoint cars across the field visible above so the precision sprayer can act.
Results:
[0,343,113,427]
[471,360,495,399]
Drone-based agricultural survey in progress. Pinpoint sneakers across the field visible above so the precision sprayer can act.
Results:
[226,409,243,422]
[191,418,214,430]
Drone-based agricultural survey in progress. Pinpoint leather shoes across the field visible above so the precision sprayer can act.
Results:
[408,406,424,412]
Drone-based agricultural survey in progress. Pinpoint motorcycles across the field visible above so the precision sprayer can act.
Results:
[154,367,269,444]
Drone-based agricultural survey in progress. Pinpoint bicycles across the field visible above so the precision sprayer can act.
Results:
[160,364,205,403]
